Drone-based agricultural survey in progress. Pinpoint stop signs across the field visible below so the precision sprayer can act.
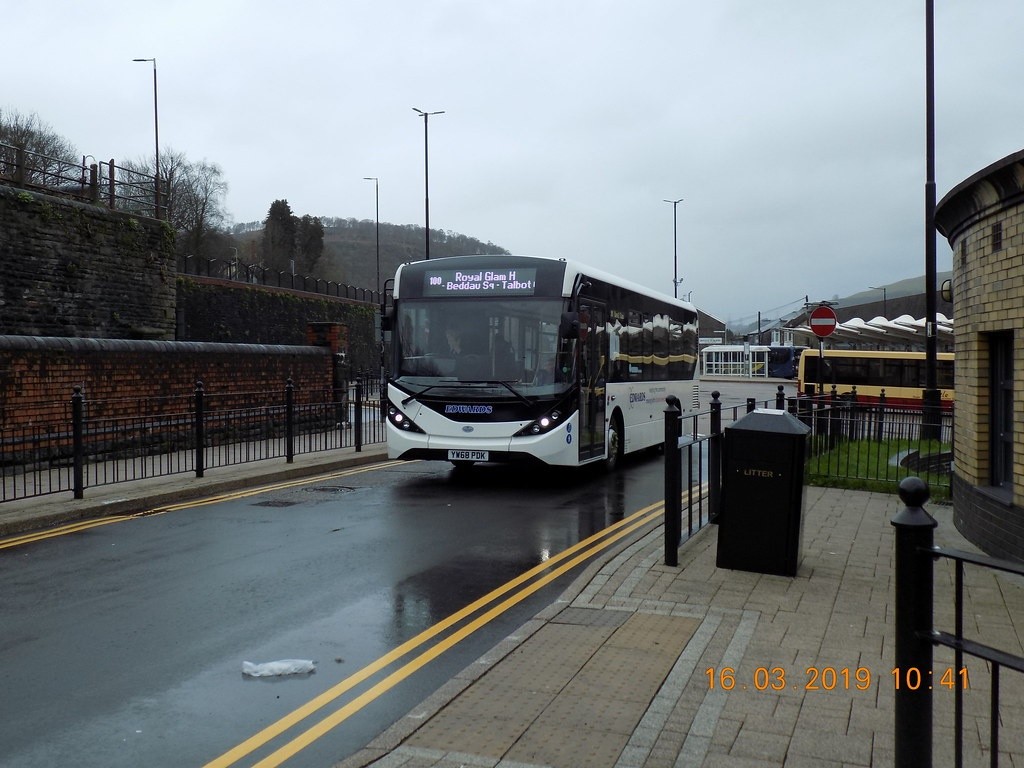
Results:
[809,306,838,338]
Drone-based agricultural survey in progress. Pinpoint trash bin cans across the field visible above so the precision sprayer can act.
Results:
[714,407,813,577]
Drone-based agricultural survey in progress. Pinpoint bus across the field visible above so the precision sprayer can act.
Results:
[703,344,811,378]
[797,348,955,412]
[380,252,700,480]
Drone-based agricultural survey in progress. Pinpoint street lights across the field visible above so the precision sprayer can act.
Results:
[364,178,380,304]
[133,58,161,219]
[413,108,446,260]
[877,287,887,317]
[663,198,684,299]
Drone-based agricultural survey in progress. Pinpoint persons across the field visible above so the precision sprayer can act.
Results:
[539,358,565,385]
[218,256,272,284]
[438,322,475,376]
